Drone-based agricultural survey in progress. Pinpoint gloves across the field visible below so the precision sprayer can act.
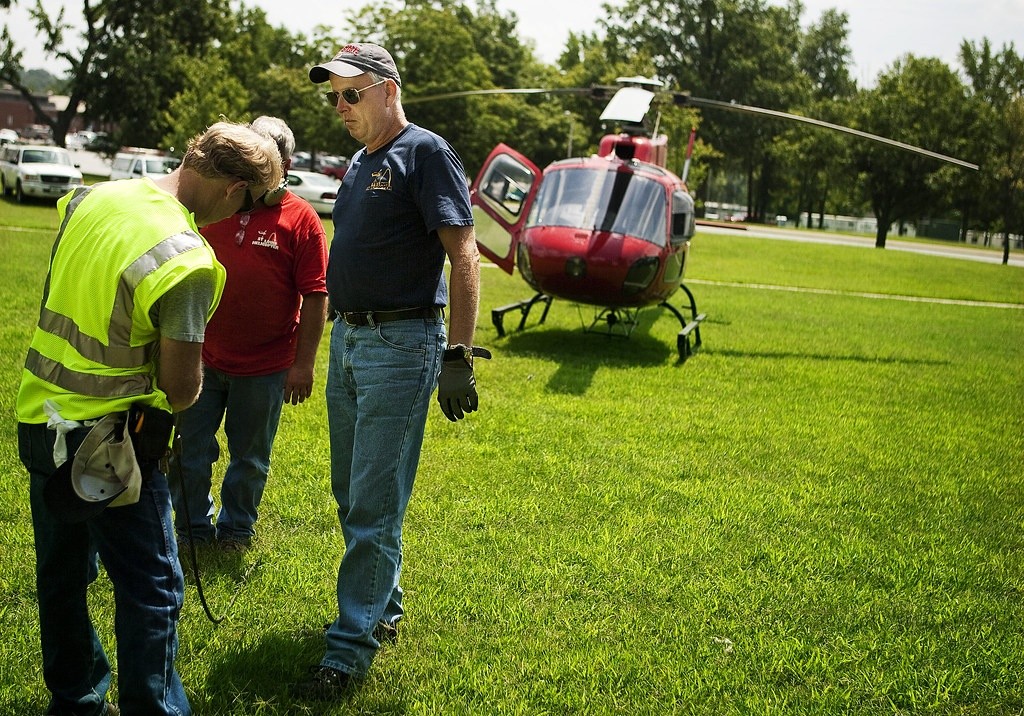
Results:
[437,347,492,422]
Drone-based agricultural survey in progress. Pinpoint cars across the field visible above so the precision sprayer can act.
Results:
[76,132,95,147]
[0,144,84,203]
[21,124,52,139]
[286,170,342,214]
[290,150,349,181]
[110,154,182,180]
[724,213,744,222]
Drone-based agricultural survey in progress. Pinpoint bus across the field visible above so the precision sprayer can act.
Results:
[704,201,749,220]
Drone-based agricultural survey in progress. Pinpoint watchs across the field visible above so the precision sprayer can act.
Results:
[447,343,492,360]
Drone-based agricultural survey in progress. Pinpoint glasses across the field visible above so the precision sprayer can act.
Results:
[235,186,255,214]
[235,214,250,246]
[326,79,390,107]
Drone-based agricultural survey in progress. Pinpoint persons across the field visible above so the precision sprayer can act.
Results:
[15,43,480,716]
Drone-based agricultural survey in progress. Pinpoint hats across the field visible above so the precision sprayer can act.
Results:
[71,411,142,507]
[309,42,402,87]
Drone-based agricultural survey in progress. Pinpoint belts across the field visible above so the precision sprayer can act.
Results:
[337,308,436,326]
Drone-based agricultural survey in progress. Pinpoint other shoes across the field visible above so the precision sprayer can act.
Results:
[177,538,213,553]
[104,704,119,716]
[323,621,397,641]
[291,665,363,698]
[219,539,251,552]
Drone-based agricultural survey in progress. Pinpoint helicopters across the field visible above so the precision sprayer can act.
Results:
[403,75,980,359]
